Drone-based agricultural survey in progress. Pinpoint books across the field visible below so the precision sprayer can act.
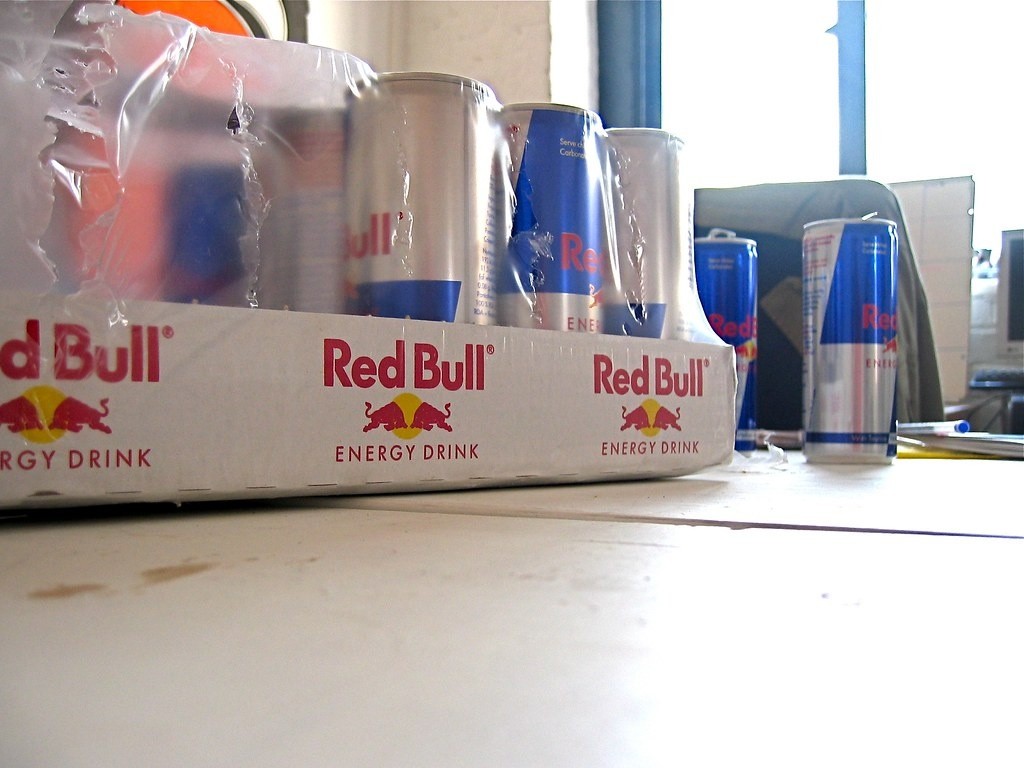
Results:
[897,433,1024,458]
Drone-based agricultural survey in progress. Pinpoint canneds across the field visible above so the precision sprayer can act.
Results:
[694,228,760,455]
[158,70,692,340]
[801,210,898,465]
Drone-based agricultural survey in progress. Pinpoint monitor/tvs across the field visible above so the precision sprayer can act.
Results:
[996,230,1024,358]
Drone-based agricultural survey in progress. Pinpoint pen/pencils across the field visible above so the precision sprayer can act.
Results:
[754,430,926,448]
[898,420,970,434]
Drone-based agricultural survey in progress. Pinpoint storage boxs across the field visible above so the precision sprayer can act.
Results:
[0,288,739,512]
